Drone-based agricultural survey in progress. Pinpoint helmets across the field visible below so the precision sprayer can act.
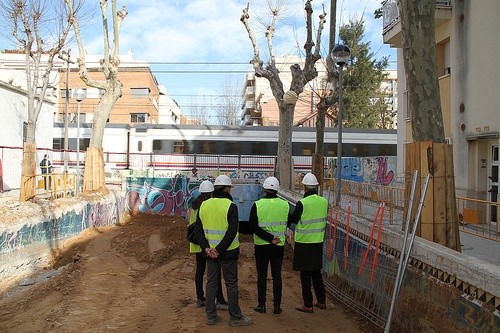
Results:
[198,180,215,193]
[213,175,232,186]
[262,176,280,192]
[301,172,319,188]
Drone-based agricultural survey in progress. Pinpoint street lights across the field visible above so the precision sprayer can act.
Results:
[283,90,298,191]
[72,87,86,197]
[331,44,352,206]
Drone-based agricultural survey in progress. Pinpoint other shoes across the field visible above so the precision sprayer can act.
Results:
[274,308,282,314]
[253,306,266,313]
[216,301,228,310]
[295,305,314,313]
[228,315,253,327]
[194,299,206,308]
[313,302,326,309]
[207,314,222,325]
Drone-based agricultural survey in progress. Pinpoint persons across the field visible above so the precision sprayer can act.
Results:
[195,175,252,327]
[250,177,291,314]
[189,180,229,310]
[291,173,329,313]
[40,154,52,190]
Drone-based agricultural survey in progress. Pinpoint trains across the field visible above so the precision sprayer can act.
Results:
[51,122,397,187]
